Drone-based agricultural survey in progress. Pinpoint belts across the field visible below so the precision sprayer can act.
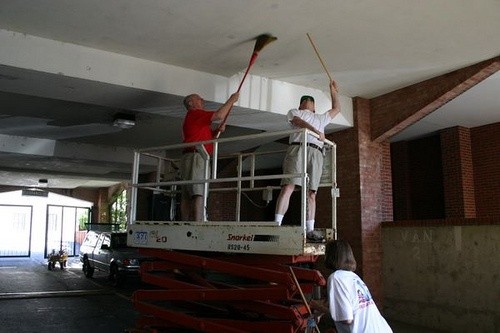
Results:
[290,142,322,152]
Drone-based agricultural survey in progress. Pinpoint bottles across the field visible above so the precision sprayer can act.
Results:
[307,314,316,333]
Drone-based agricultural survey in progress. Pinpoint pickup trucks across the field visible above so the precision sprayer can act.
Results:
[79,223,157,288]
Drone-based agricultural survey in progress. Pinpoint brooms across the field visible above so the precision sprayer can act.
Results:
[214,33,278,138]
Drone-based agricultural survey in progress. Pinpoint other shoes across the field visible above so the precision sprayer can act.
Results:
[307,231,326,243]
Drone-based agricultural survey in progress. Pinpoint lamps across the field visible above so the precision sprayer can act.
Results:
[112,112,136,128]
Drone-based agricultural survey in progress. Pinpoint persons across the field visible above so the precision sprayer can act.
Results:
[310,240,393,333]
[275,80,342,242]
[180,93,240,221]
